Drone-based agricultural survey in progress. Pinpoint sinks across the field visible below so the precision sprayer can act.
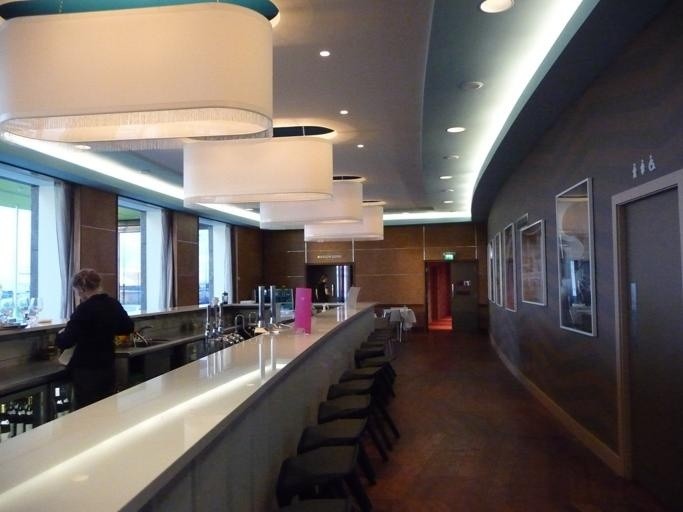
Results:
[129,341,157,348]
[150,338,169,342]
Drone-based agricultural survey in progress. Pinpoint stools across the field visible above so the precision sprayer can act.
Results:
[276,328,399,512]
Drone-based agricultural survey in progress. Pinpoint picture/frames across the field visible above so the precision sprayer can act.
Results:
[487,178,598,338]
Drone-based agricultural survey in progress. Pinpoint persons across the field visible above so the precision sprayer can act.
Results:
[315,273,331,303]
[53,267,134,409]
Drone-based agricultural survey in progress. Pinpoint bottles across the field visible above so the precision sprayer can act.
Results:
[53,386,71,419]
[0,396,33,442]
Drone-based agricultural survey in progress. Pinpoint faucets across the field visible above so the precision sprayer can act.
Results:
[133,324,155,340]
[247,310,257,323]
[234,311,247,333]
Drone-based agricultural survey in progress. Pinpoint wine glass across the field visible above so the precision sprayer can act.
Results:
[0,290,44,328]
